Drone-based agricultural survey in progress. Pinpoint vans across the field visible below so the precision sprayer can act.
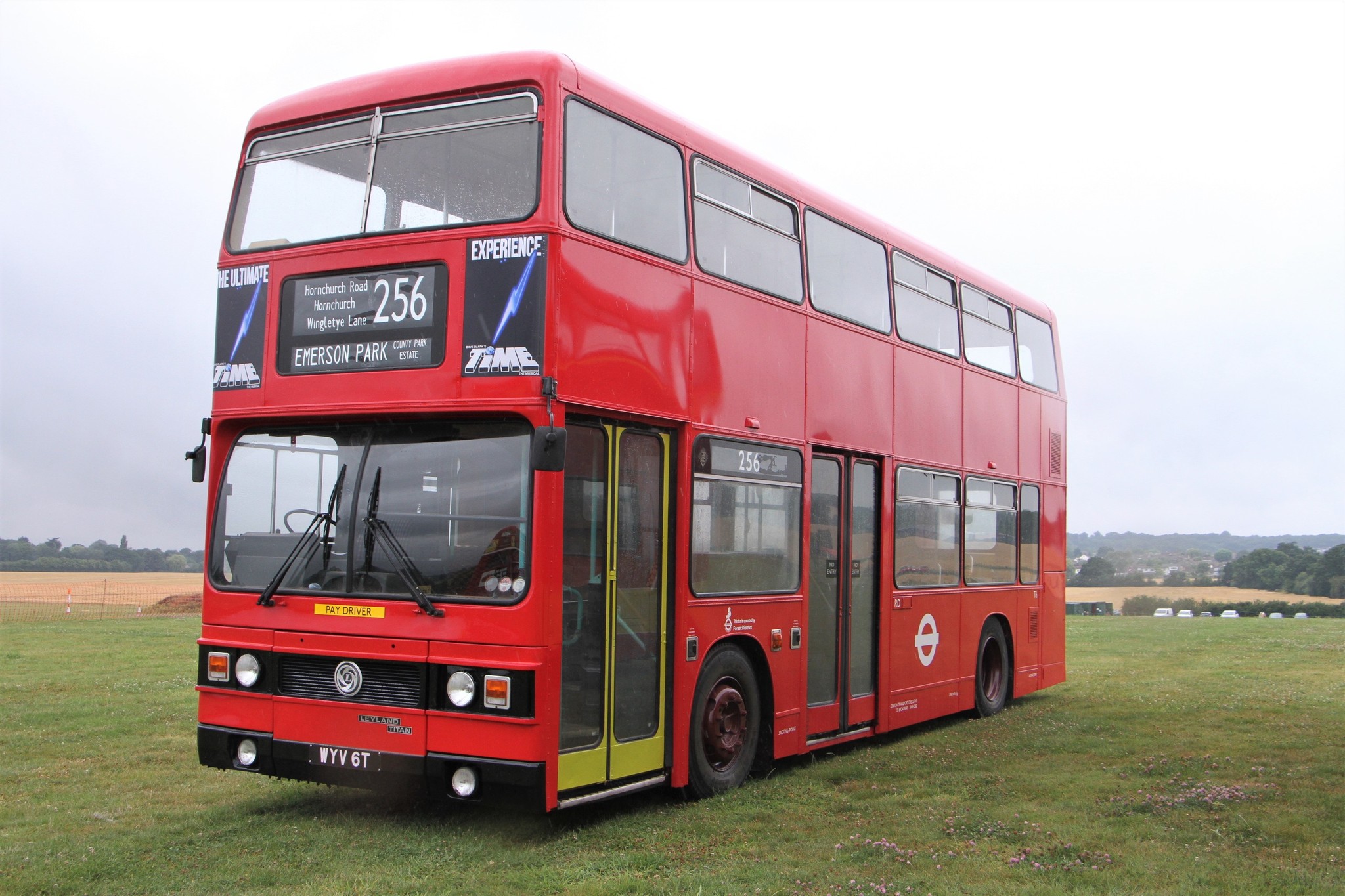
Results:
[1293,613,1309,619]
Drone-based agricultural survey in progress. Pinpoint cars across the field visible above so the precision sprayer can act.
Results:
[1153,608,1173,617]
[1113,610,1123,616]
[1177,610,1194,617]
[1267,613,1284,618]
[1200,612,1215,617]
[1220,610,1239,618]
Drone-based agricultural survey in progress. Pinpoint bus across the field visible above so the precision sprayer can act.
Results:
[184,50,1068,813]
